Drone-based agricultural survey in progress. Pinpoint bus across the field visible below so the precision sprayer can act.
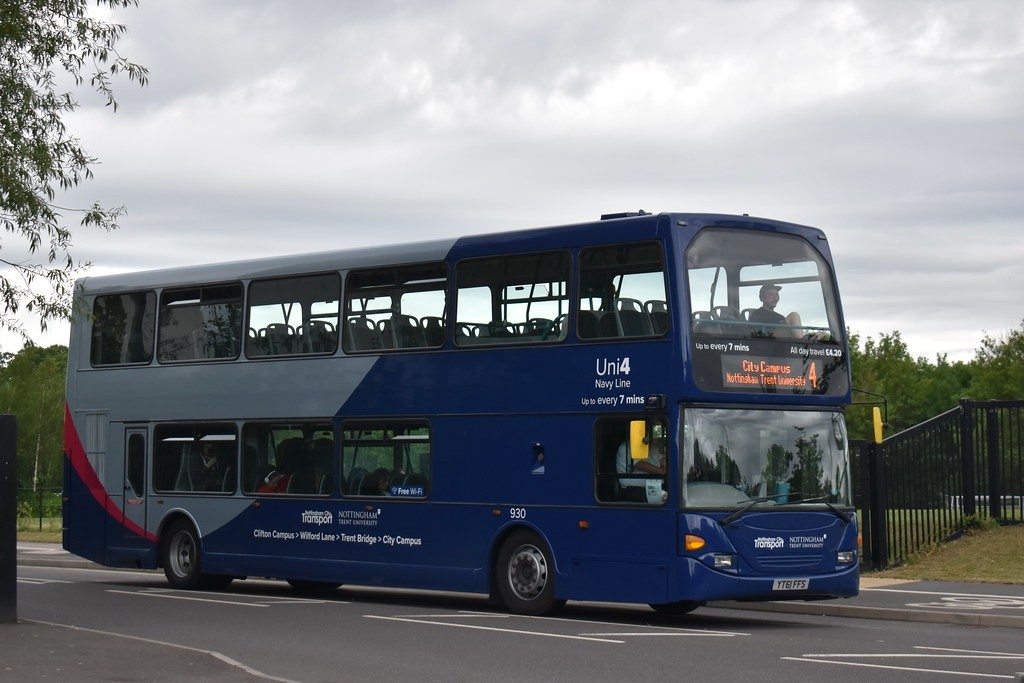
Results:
[59,219,889,615]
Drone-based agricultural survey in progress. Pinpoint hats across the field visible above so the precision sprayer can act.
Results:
[759,283,782,302]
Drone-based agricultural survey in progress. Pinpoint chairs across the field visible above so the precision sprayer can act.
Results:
[173,442,413,495]
[193,299,758,359]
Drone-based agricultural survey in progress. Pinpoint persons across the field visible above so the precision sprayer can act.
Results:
[615,416,667,488]
[369,468,392,496]
[256,466,290,493]
[189,440,227,491]
[747,287,804,339]
